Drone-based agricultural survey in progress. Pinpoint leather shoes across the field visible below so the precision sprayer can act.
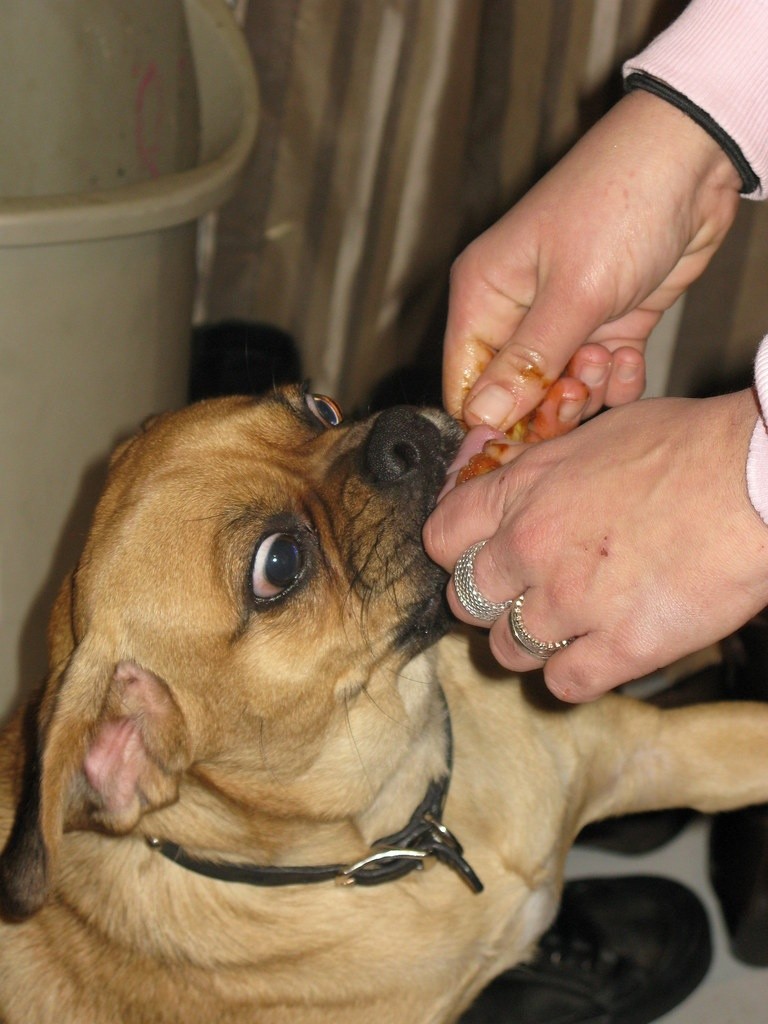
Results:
[458,875,712,1024]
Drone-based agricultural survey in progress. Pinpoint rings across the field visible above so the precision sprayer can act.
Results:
[453,533,514,622]
[507,589,579,660]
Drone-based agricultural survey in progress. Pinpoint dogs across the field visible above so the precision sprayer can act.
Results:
[0,377,768,1024]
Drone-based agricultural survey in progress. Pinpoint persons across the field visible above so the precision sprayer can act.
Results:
[420,0,768,712]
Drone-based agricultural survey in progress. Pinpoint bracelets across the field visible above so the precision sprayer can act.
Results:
[625,71,760,198]
